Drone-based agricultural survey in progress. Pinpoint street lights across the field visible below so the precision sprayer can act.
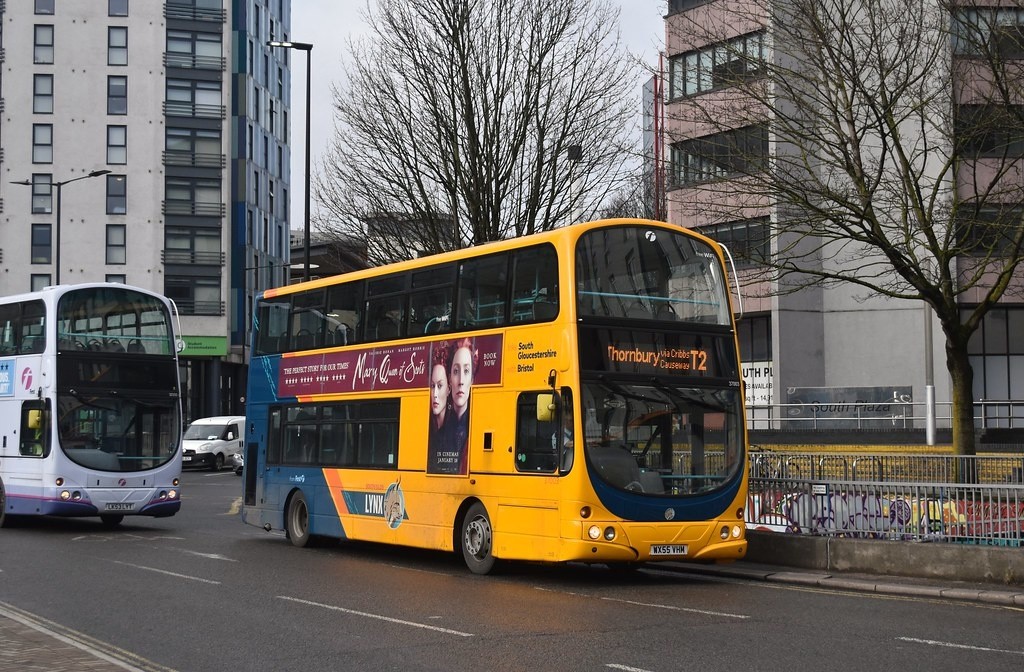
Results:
[9,169,113,287]
[266,40,313,282]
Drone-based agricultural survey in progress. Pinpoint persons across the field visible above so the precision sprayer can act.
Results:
[551,410,574,467]
[427,337,478,473]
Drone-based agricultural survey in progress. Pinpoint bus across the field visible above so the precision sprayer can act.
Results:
[0,281,184,528]
[241,218,748,575]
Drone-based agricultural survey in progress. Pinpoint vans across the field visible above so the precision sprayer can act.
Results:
[182,416,245,473]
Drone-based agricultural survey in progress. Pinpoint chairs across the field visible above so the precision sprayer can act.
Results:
[277,315,418,351]
